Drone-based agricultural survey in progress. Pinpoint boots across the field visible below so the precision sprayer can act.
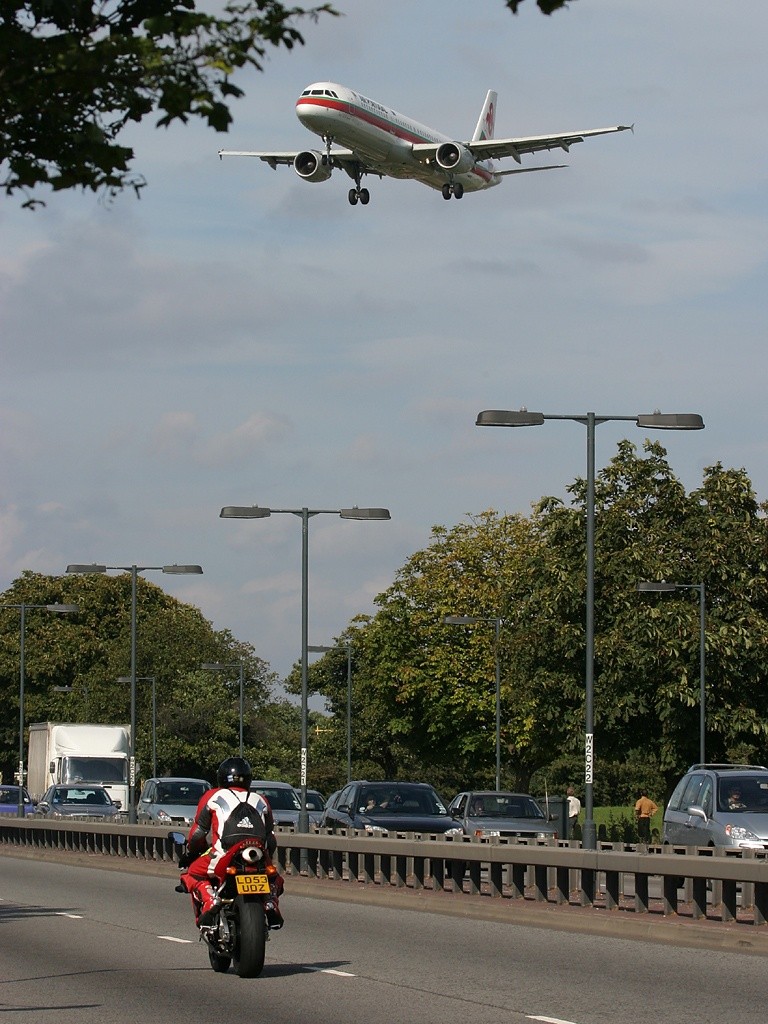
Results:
[264,881,284,930]
[195,879,221,928]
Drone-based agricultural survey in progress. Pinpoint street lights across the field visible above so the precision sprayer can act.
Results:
[306,645,352,785]
[55,686,88,724]
[475,406,706,902]
[444,616,501,805]
[201,662,243,759]
[635,581,705,769]
[219,504,391,871]
[64,563,204,824]
[117,678,157,778]
[0,603,82,836]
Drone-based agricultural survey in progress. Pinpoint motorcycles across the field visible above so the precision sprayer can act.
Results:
[167,830,282,978]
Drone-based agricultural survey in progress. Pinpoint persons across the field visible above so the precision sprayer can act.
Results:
[567,787,581,838]
[470,798,486,817]
[726,783,747,809]
[359,791,390,812]
[187,758,284,931]
[635,790,658,843]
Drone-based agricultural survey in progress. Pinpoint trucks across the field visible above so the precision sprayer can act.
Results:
[26,722,141,822]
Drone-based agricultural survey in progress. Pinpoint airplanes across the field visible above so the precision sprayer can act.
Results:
[218,80,635,206]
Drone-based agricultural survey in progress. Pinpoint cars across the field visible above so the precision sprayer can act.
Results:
[35,783,123,844]
[446,789,560,846]
[248,778,329,831]
[0,785,38,819]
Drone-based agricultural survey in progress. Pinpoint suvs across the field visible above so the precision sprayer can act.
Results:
[321,780,466,879]
[136,776,213,824]
[662,763,768,892]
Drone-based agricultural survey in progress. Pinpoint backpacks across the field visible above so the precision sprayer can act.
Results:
[217,786,266,850]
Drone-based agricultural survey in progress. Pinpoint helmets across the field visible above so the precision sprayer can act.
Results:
[216,756,252,790]
